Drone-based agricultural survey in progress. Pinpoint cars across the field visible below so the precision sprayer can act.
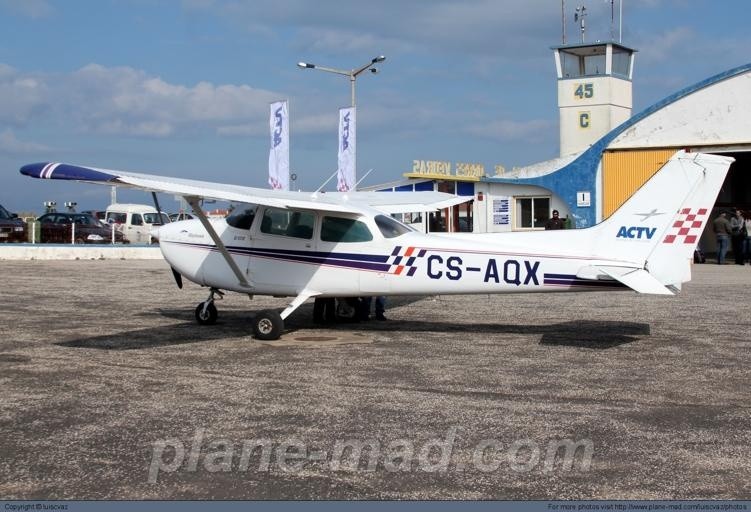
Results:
[0,203,198,244]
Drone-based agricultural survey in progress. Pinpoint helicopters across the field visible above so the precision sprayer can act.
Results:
[20,150,735,340]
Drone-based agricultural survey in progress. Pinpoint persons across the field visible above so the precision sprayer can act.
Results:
[713,210,733,264]
[743,212,751,265]
[730,207,746,266]
[545,209,565,231]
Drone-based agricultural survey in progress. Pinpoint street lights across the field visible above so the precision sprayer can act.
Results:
[297,54,386,108]
